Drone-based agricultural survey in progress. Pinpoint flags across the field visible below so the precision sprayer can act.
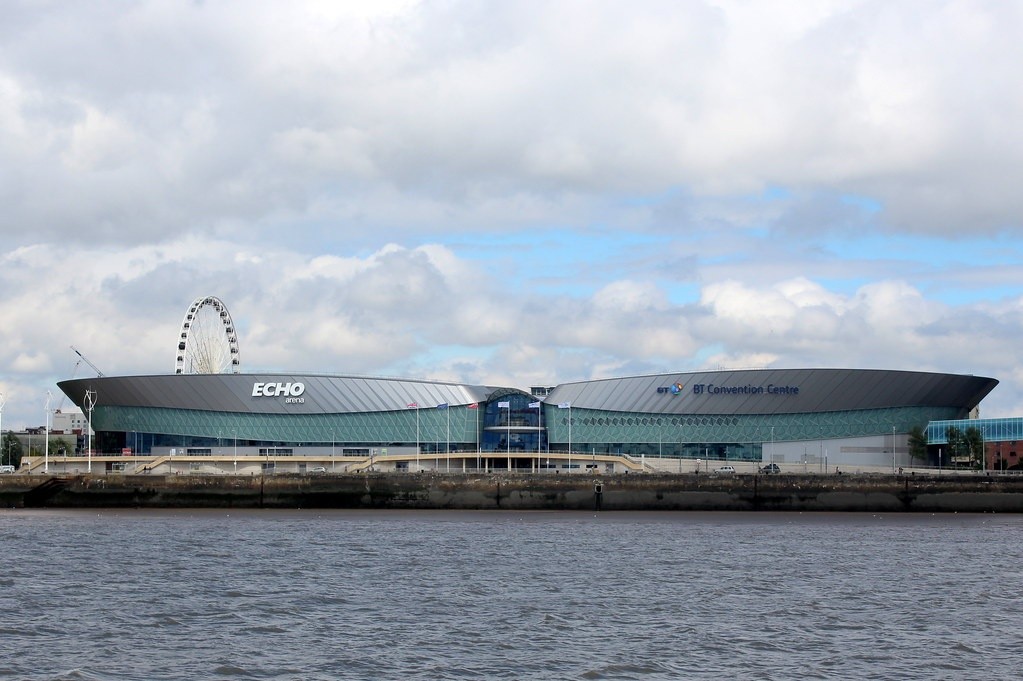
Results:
[498,402,509,407]
[437,403,449,410]
[558,401,571,408]
[529,402,540,408]
[468,403,478,408]
[407,402,417,408]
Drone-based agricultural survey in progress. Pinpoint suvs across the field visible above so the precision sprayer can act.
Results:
[714,465,736,474]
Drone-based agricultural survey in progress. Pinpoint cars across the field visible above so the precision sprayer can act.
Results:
[758,464,781,474]
[310,466,327,474]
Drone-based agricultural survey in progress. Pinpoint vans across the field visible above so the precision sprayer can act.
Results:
[0,465,16,474]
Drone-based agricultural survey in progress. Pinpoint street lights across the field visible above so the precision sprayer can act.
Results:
[231,429,237,473]
[329,427,336,473]
[771,427,775,475]
[8,441,18,466]
[680,424,683,472]
[131,429,138,470]
[545,426,551,470]
[892,425,897,473]
[982,426,986,474]
